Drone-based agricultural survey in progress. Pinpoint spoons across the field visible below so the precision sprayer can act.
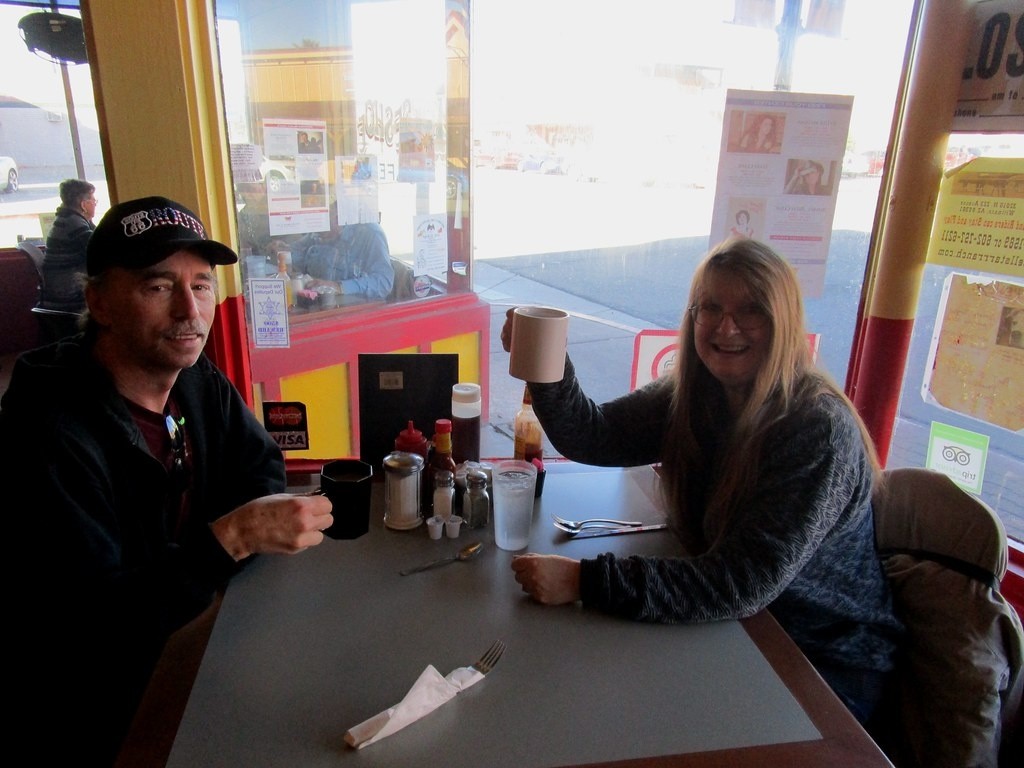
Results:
[398,541,485,576]
[552,521,632,533]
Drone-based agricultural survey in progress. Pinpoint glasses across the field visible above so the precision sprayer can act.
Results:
[688,305,769,330]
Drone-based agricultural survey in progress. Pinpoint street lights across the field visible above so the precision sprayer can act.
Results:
[724,0,845,93]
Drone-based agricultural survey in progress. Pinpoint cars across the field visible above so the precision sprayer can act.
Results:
[258,157,292,193]
[473,145,568,175]
[0,156,19,193]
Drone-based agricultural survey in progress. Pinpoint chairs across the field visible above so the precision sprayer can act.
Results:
[20,239,89,348]
[390,256,416,302]
[873,468,1024,768]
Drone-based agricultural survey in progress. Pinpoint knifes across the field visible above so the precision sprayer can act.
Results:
[570,524,669,539]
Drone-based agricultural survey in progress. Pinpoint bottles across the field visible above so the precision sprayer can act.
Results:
[289,270,305,304]
[430,419,456,496]
[451,382,480,465]
[433,471,457,525]
[395,420,428,475]
[276,265,292,307]
[462,471,489,529]
[383,452,425,530]
[514,385,542,469]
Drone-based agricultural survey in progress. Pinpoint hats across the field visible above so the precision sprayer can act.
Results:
[85,195,237,273]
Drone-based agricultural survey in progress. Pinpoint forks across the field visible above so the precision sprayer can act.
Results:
[469,639,508,677]
[550,512,642,528]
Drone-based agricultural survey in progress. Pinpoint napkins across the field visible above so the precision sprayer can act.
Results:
[345,663,486,750]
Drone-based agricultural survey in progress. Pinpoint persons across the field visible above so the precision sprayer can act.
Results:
[230,165,395,308]
[720,209,755,249]
[298,132,313,153]
[730,106,786,154]
[498,236,909,762]
[39,179,98,329]
[781,157,830,196]
[0,194,335,768]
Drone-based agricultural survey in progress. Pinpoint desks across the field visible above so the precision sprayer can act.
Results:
[106,457,899,768]
[248,262,386,327]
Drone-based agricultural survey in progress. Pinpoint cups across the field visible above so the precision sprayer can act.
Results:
[426,515,445,539]
[312,457,374,540]
[444,515,462,538]
[508,306,569,383]
[491,461,536,551]
